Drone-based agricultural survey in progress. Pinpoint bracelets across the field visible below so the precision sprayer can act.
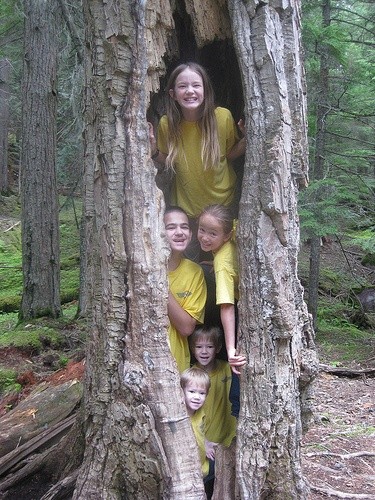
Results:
[151,149,160,158]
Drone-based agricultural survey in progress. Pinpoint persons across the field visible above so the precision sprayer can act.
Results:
[147,62,246,264]
[191,324,238,449]
[180,368,219,477]
[198,204,247,417]
[164,205,207,374]
